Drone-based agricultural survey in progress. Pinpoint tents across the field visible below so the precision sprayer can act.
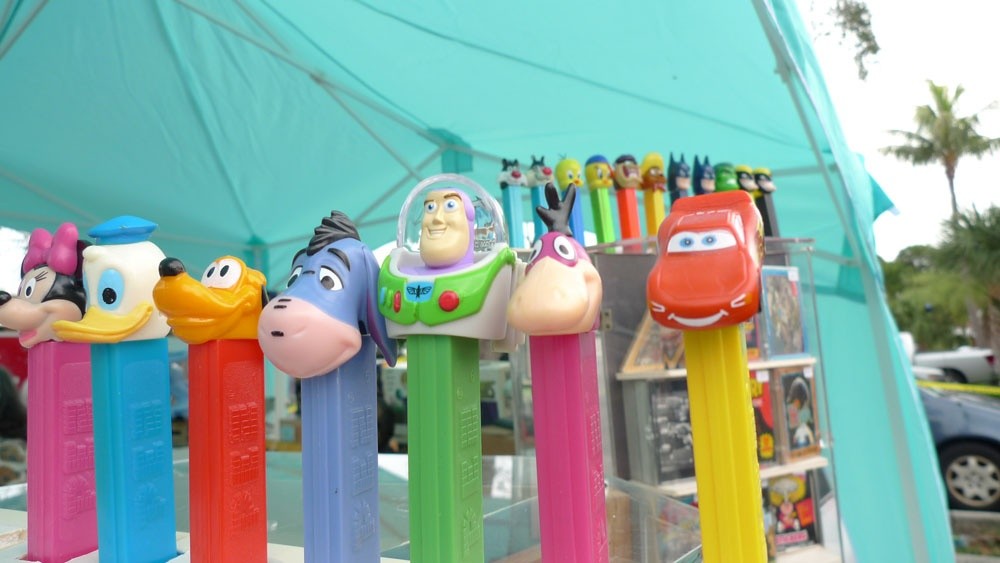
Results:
[0,0,957,563]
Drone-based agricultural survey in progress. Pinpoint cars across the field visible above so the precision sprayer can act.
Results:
[913,348,999,511]
